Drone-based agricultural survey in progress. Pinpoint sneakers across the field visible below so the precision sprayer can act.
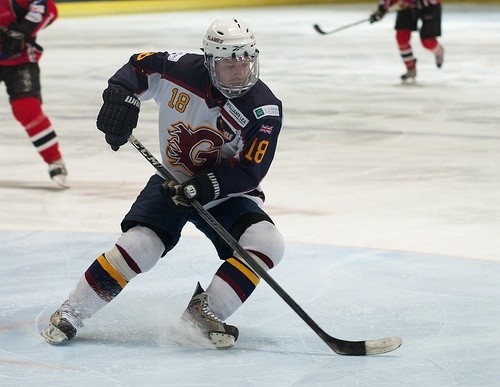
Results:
[436,48,445,67]
[181,282,239,349]
[401,59,417,84]
[47,160,70,188]
[41,301,85,344]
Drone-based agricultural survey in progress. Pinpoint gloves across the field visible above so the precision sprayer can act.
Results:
[97,88,141,151]
[3,24,25,55]
[415,0,431,9]
[160,169,220,207]
[371,4,386,23]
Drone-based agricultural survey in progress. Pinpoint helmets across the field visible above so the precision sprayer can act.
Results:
[203,18,259,99]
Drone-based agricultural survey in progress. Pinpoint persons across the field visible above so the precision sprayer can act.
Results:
[0,0,72,188]
[370,0,444,82]
[38,16,286,350]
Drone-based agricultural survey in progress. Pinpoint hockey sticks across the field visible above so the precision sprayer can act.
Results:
[125,133,406,356]
[315,18,374,35]
